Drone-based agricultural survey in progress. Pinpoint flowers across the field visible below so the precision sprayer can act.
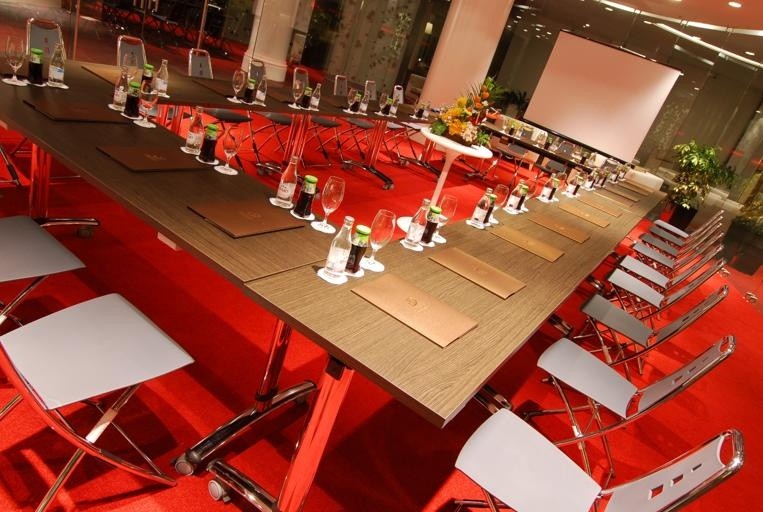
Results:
[428,75,506,141]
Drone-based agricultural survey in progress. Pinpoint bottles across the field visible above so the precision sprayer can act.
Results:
[309,82,323,110]
[256,74,269,105]
[48,41,66,86]
[184,105,205,155]
[349,93,362,113]
[154,57,172,97]
[381,98,394,116]
[274,156,300,208]
[472,105,629,196]
[403,198,432,249]
[140,63,155,94]
[420,205,442,245]
[506,178,524,211]
[469,187,494,228]
[415,101,426,120]
[360,90,371,114]
[302,86,312,109]
[27,47,44,87]
[516,185,528,211]
[123,79,142,120]
[344,225,372,274]
[483,193,499,225]
[538,173,556,202]
[293,174,317,220]
[424,99,431,123]
[197,124,219,164]
[390,92,402,116]
[112,65,127,110]
[244,76,257,104]
[321,214,355,279]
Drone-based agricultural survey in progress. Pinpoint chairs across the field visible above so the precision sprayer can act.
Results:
[0,213,87,329]
[652,209,725,239]
[648,215,723,248]
[453,408,745,512]
[0,291,195,512]
[613,244,726,296]
[606,257,728,331]
[616,231,723,275]
[27,16,68,59]
[0,144,23,190]
[572,284,729,381]
[480,123,617,191]
[638,222,723,258]
[522,334,736,512]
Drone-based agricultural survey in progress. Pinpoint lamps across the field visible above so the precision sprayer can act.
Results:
[673,18,734,66]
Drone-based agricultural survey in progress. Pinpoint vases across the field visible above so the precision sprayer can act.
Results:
[441,128,472,146]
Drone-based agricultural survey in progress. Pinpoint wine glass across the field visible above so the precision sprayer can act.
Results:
[375,90,388,116]
[137,78,158,127]
[361,208,397,267]
[411,97,422,118]
[231,70,246,103]
[432,194,458,240]
[346,87,359,112]
[290,79,305,108]
[220,124,242,174]
[554,171,568,200]
[4,34,28,85]
[121,50,140,88]
[317,174,346,230]
[521,177,538,207]
[489,183,509,222]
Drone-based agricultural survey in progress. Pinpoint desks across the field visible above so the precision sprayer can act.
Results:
[0,67,347,281]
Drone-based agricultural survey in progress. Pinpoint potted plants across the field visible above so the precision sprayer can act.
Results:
[715,198,763,276]
[667,139,740,231]
[506,91,529,119]
